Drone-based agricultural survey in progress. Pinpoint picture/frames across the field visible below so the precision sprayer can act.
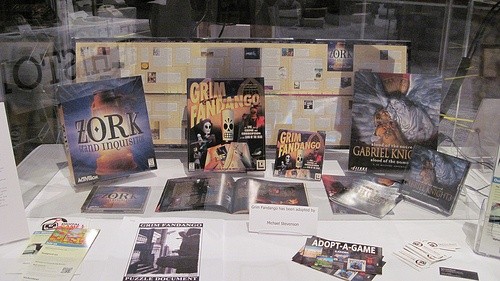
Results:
[480,44,500,82]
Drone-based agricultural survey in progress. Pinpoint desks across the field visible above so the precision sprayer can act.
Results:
[271,26,396,40]
[0,17,151,123]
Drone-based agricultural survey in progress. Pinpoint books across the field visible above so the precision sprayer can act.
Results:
[155,173,310,214]
[122,222,203,281]
[185,76,266,177]
[53,75,158,186]
[273,71,472,219]
[81,185,152,214]
[4,226,100,281]
